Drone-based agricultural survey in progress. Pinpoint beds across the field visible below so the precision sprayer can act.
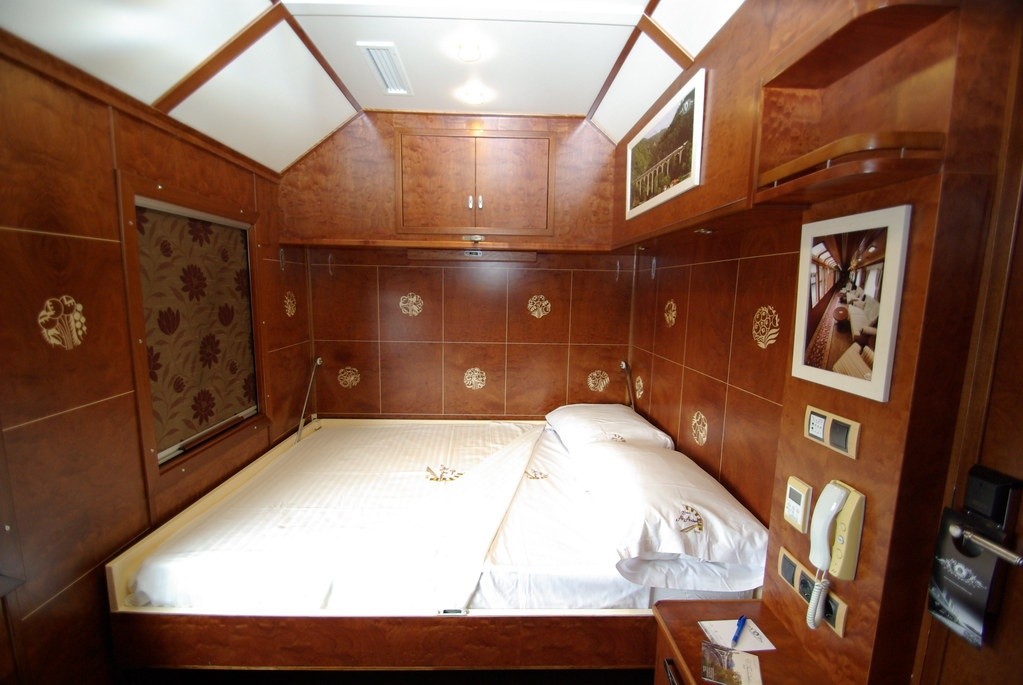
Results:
[104,415,765,685]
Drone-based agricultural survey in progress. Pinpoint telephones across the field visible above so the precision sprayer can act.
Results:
[808,479,865,581]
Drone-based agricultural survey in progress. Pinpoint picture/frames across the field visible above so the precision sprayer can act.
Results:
[791,202,913,403]
[623,67,707,221]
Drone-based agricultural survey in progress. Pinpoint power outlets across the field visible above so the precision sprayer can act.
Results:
[807,411,827,443]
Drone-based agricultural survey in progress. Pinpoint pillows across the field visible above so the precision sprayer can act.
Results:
[543,401,674,455]
[577,448,769,590]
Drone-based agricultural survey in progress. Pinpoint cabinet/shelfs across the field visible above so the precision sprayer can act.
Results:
[394,127,556,236]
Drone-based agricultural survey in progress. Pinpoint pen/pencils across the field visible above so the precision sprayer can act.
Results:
[732,615,746,648]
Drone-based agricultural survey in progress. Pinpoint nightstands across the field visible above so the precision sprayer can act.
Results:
[651,598,836,685]
